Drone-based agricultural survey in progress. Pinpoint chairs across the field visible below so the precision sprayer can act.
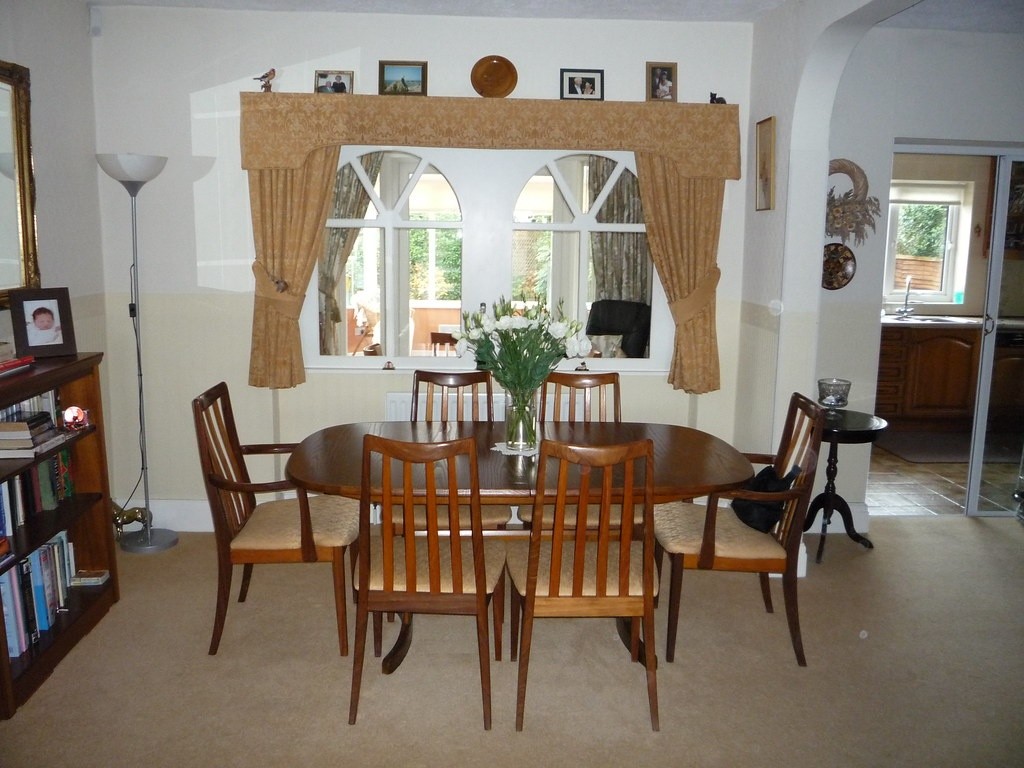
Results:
[585,300,650,358]
[189,380,364,659]
[511,435,657,731]
[518,368,648,532]
[383,367,493,623]
[651,391,832,664]
[346,432,507,730]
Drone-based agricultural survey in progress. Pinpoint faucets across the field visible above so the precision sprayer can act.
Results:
[894,274,915,318]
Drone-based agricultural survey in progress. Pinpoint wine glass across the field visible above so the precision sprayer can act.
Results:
[818,378,852,422]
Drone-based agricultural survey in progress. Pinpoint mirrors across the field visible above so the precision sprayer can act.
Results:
[0,59,43,310]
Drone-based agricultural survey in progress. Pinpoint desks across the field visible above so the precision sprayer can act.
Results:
[287,419,754,667]
[798,411,889,565]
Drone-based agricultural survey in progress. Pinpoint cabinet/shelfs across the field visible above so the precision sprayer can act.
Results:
[874,327,981,415]
[972,329,1024,426]
[0,351,120,722]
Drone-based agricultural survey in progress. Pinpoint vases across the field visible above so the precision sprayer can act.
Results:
[507,399,540,452]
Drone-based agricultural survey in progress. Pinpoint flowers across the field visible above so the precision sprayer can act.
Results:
[451,294,593,405]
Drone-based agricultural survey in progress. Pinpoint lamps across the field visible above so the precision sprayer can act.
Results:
[96,151,175,553]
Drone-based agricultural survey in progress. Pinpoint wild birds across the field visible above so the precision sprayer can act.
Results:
[251,68,276,84]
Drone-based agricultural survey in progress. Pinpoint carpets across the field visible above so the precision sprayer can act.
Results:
[873,428,1021,464]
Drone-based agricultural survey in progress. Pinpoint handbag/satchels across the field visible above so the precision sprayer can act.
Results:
[731,464,802,533]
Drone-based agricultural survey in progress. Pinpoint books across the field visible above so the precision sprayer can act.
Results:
[0,530,110,683]
[0,355,35,380]
[0,391,68,458]
[0,442,79,568]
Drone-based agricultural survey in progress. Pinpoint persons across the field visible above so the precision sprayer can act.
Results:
[653,68,673,100]
[27,306,63,346]
[569,77,594,94]
[322,76,346,93]
[400,75,410,92]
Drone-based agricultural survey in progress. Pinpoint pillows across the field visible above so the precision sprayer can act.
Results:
[587,335,622,357]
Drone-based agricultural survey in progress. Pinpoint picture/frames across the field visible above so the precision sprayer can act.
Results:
[379,60,429,95]
[983,154,1024,259]
[560,68,605,98]
[755,117,775,213]
[314,69,355,93]
[646,61,680,102]
[8,288,77,359]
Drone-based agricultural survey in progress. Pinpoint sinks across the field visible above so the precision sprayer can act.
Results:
[894,316,956,323]
